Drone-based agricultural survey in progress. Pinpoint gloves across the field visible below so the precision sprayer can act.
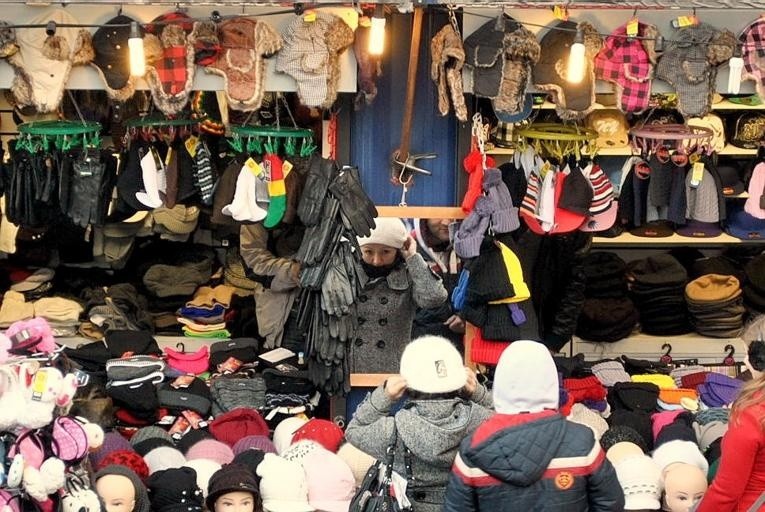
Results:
[294,155,378,400]
[4,147,117,229]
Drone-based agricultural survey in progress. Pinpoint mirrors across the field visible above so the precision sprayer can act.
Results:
[346,206,478,387]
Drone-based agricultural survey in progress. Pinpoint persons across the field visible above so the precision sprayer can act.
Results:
[347,218,448,372]
[443,340,625,512]
[344,335,494,512]
[664,464,708,512]
[214,491,254,512]
[411,219,465,354]
[96,474,135,512]
[695,315,765,512]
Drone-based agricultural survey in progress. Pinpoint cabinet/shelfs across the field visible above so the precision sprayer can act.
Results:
[545,92,765,366]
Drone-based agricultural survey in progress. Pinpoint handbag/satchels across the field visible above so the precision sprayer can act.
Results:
[348,419,416,512]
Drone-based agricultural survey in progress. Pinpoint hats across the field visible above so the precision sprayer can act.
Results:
[203,16,284,115]
[141,11,221,115]
[595,19,664,115]
[432,10,467,122]
[400,335,468,393]
[356,217,407,249]
[492,340,560,417]
[90,13,164,104]
[2,5,92,115]
[451,144,765,366]
[656,21,736,118]
[1,230,344,512]
[462,12,542,115]
[274,9,353,111]
[556,353,746,511]
[531,21,604,121]
[736,16,765,100]
